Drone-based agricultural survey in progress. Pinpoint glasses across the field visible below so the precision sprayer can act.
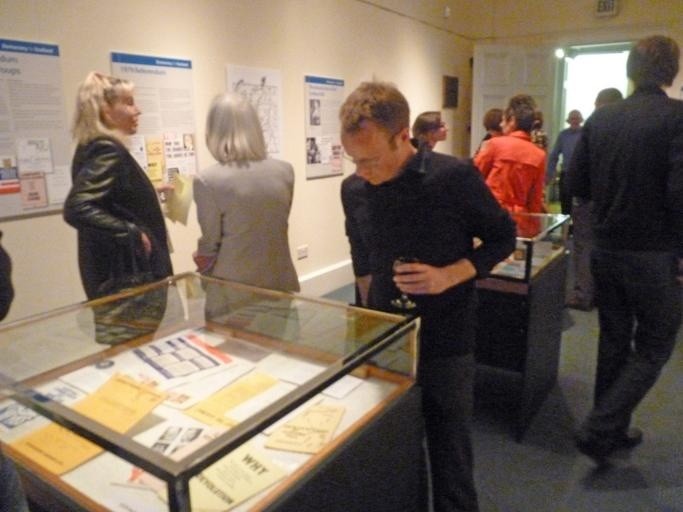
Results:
[340,146,382,168]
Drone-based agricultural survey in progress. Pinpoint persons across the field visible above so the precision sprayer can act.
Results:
[62,71,176,346]
[561,35,683,467]
[339,80,516,512]
[1,226,30,510]
[472,87,626,313]
[410,111,448,152]
[191,92,301,337]
[306,138,318,162]
[311,100,321,125]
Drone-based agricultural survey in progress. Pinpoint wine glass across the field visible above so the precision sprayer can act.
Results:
[390,257,420,309]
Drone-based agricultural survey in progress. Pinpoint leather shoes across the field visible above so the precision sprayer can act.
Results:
[575,426,642,472]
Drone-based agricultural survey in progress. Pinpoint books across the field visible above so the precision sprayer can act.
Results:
[0,329,363,511]
